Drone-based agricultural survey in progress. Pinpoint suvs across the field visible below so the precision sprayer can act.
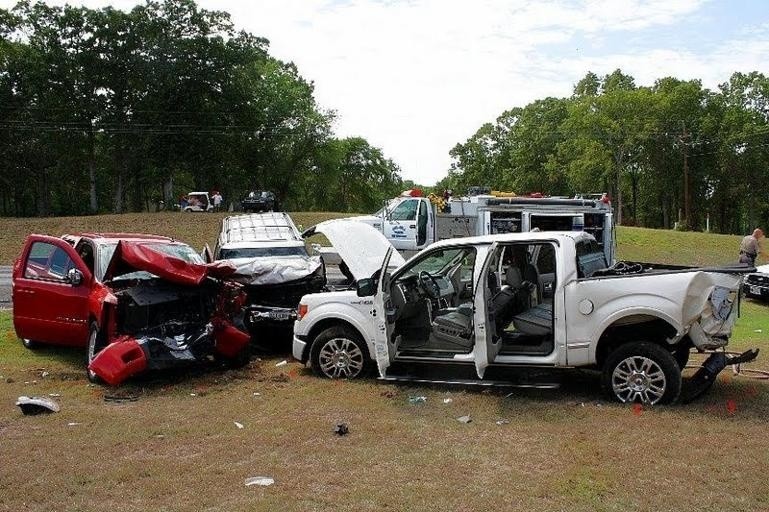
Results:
[242,190,280,213]
[197,208,327,339]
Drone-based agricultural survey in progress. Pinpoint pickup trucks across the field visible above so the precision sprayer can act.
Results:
[281,228,754,408]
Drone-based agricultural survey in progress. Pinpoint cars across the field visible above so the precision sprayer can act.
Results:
[739,258,769,303]
[13,227,249,384]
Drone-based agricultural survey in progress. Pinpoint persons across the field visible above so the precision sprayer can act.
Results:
[211,191,223,212]
[739,228,769,269]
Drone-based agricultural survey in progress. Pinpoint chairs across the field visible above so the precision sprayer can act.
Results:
[429,244,554,351]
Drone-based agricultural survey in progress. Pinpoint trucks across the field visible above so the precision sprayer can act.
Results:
[309,185,621,279]
